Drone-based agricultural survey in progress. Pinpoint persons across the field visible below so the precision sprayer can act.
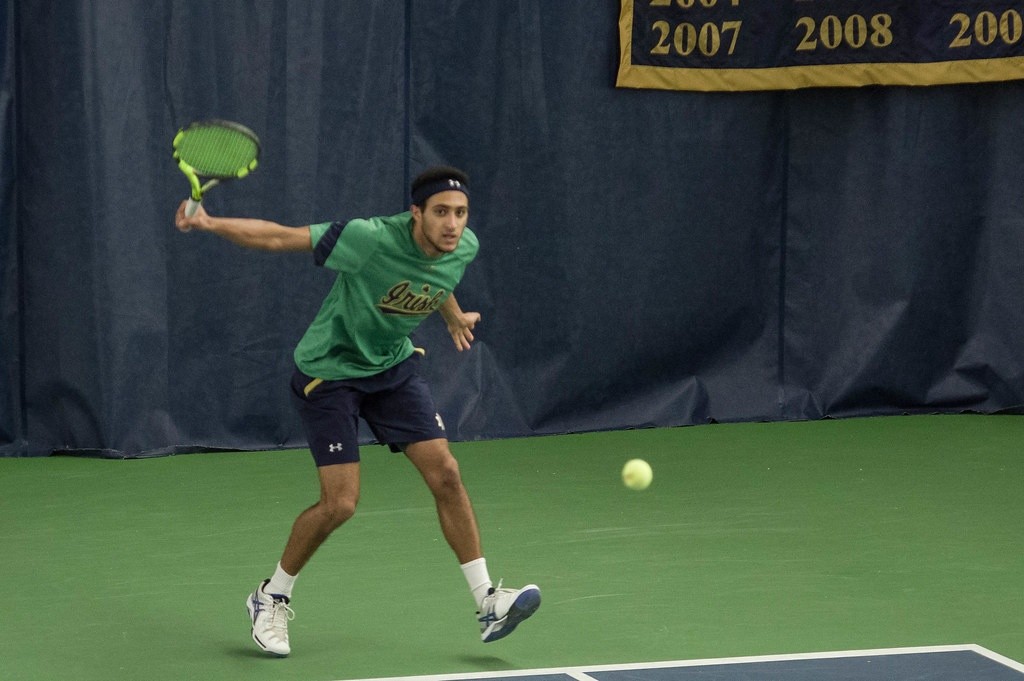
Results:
[175,169,543,658]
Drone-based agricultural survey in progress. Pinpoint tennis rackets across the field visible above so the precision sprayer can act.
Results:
[169,115,262,222]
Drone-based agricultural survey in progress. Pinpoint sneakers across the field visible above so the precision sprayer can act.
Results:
[477,576,542,643]
[246,577,296,655]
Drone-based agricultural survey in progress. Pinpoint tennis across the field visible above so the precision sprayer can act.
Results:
[621,458,654,491]
